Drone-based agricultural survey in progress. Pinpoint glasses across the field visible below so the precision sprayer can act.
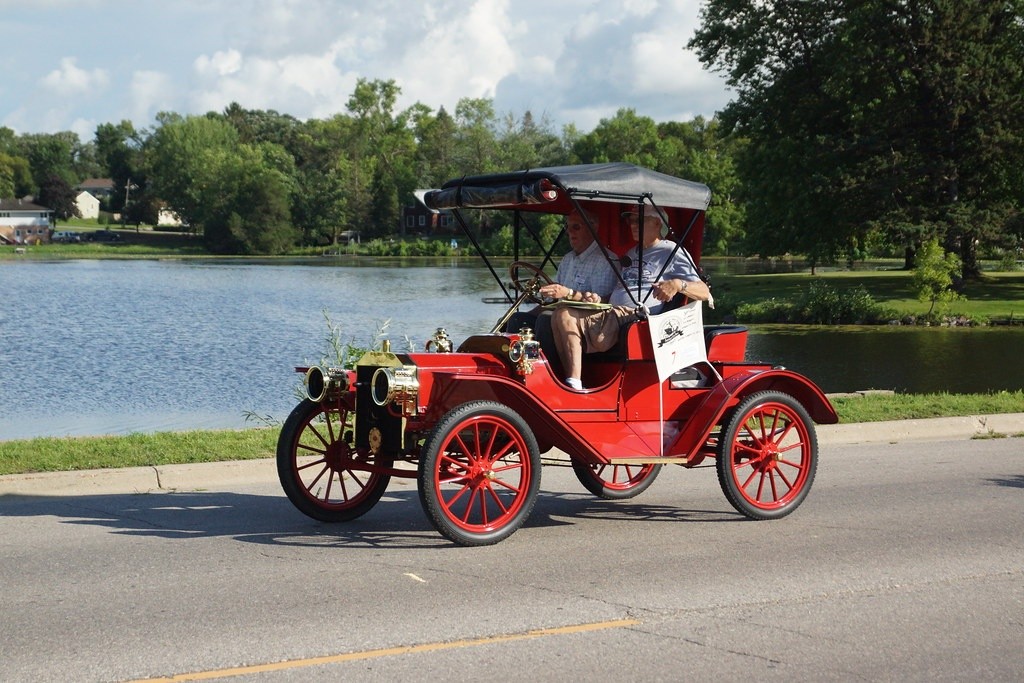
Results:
[563,224,580,230]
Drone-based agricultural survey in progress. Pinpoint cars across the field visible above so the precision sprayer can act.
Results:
[273,166,839,548]
[51,229,121,243]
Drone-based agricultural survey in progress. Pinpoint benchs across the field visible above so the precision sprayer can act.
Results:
[499,293,688,362]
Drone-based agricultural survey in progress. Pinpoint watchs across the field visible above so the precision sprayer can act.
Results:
[566,289,577,300]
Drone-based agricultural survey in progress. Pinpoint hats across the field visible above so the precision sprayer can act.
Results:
[621,204,669,238]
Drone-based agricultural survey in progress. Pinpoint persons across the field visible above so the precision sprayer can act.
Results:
[551,205,709,390]
[507,208,621,374]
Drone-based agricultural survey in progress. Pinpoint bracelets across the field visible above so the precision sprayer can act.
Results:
[680,280,687,294]
